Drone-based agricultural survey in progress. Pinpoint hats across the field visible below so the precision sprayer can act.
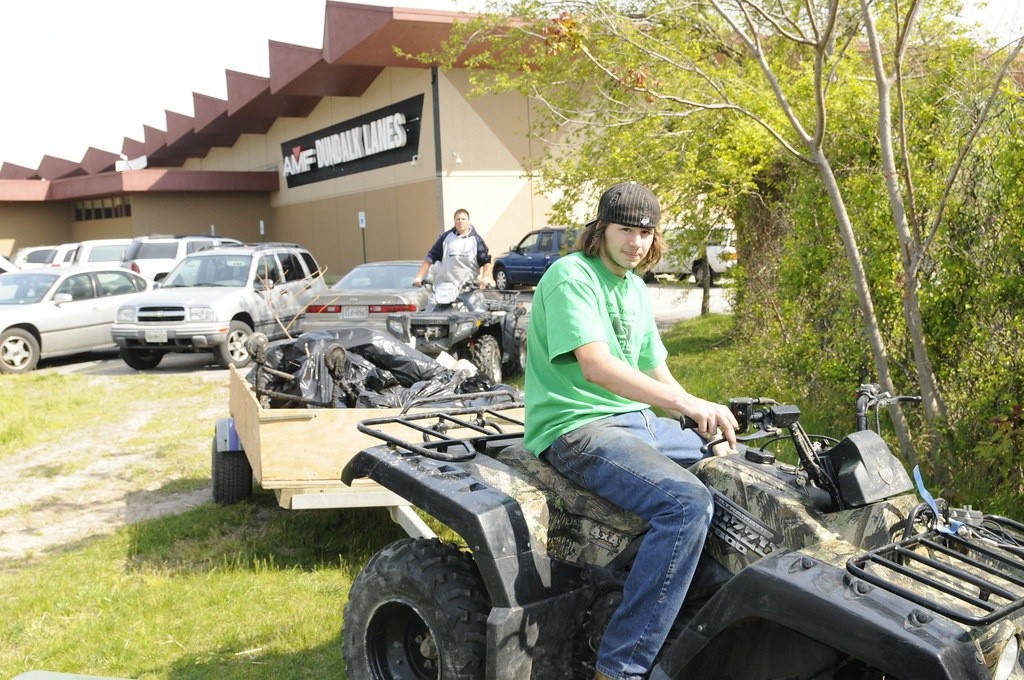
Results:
[583,181,662,229]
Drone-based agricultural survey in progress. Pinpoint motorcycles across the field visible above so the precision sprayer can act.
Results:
[339,379,1024,680]
[385,279,527,385]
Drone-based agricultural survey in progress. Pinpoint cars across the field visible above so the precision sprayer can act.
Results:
[642,225,738,288]
[1,256,37,301]
[0,268,161,375]
[492,227,581,293]
[13,246,60,269]
[300,260,438,344]
[71,239,133,272]
[45,243,81,270]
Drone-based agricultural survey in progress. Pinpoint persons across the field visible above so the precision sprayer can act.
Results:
[413,209,492,313]
[522,181,740,680]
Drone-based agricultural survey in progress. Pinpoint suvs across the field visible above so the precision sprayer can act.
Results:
[121,234,248,284]
[110,243,329,371]
